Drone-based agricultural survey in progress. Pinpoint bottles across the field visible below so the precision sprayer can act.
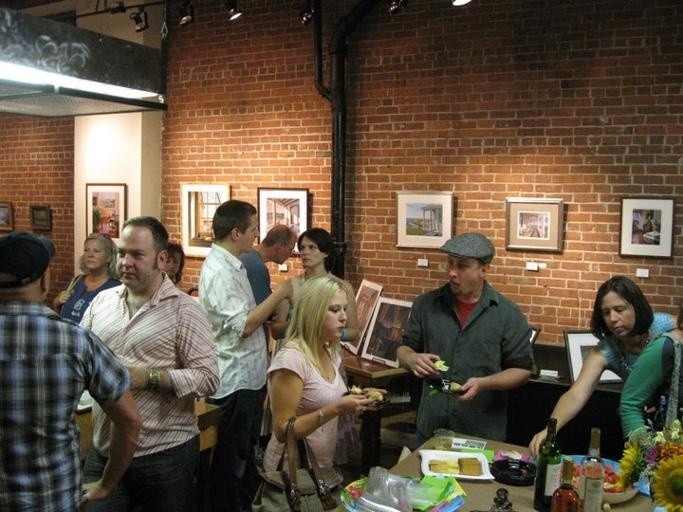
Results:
[534,418,561,512]
[580,427,604,512]
[550,456,580,512]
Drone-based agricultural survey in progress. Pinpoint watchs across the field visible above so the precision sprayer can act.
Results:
[148,368,159,390]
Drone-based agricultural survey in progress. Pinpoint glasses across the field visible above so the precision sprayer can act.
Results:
[287,245,296,255]
[90,232,112,240]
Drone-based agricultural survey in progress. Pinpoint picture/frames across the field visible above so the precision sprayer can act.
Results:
[179,180,232,259]
[530,327,541,344]
[85,183,128,248]
[504,196,564,254]
[360,297,413,369]
[395,190,455,250]
[257,187,310,257]
[31,205,51,231]
[338,278,384,357]
[563,328,626,385]
[0,202,14,232]
[619,197,675,263]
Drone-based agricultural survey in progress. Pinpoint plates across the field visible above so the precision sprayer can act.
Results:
[358,392,388,411]
[418,448,495,480]
[427,378,461,392]
[417,435,486,460]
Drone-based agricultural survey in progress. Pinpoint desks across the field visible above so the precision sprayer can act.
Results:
[336,345,422,455]
[508,342,625,462]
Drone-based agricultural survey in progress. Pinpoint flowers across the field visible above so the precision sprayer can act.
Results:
[616,417,683,512]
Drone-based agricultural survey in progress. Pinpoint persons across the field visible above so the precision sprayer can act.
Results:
[164,243,184,285]
[0,228,141,512]
[76,217,219,512]
[530,273,683,460]
[238,223,297,336]
[196,201,293,511]
[619,299,683,447]
[268,228,359,341]
[262,274,389,478]
[52,231,123,324]
[393,234,535,448]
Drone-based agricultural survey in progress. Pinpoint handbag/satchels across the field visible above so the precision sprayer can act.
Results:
[251,416,343,512]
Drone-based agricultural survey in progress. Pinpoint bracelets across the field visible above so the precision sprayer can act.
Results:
[318,407,323,427]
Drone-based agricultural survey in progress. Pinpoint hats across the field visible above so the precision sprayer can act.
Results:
[0,230,56,288]
[440,232,495,264]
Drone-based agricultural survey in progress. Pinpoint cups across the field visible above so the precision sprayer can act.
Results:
[433,429,454,450]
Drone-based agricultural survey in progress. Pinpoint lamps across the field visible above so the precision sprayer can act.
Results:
[176,2,193,26]
[387,1,402,15]
[129,6,149,33]
[223,1,243,22]
[298,6,318,26]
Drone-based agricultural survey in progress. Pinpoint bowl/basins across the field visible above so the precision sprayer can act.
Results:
[561,456,639,503]
[490,459,537,486]
[342,475,463,511]
